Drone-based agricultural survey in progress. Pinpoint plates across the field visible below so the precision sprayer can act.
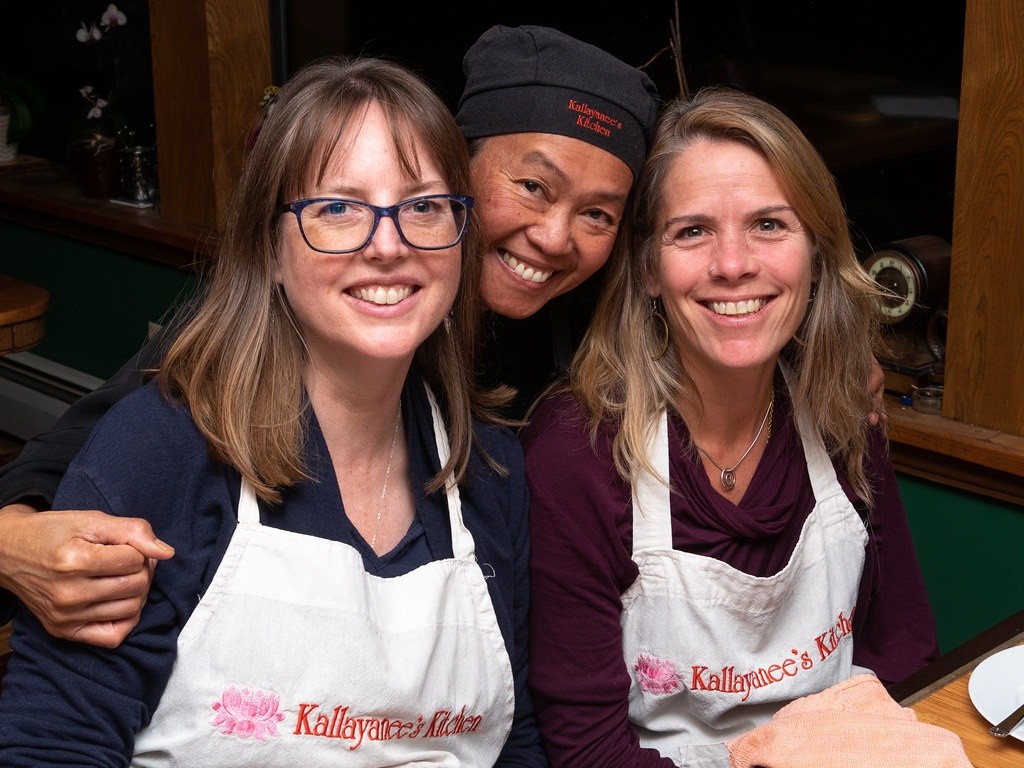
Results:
[968,644,1024,745]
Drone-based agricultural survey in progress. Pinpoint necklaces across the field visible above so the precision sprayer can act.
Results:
[369,399,401,546]
[681,385,775,492]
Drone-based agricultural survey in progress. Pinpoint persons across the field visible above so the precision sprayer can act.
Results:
[0,23,887,651]
[0,58,542,768]
[523,90,938,768]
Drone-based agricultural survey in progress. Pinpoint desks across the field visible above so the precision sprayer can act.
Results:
[659,57,960,170]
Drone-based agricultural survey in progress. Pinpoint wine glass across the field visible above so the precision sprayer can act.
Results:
[118,124,156,203]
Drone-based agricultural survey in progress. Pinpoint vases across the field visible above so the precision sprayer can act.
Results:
[77,144,124,200]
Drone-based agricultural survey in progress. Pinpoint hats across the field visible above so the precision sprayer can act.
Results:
[455,24,662,184]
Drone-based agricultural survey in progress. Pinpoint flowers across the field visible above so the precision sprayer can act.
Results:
[74,3,127,149]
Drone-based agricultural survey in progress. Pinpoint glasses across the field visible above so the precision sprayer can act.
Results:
[278,193,476,254]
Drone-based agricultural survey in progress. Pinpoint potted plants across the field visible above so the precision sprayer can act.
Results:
[0,73,39,161]
